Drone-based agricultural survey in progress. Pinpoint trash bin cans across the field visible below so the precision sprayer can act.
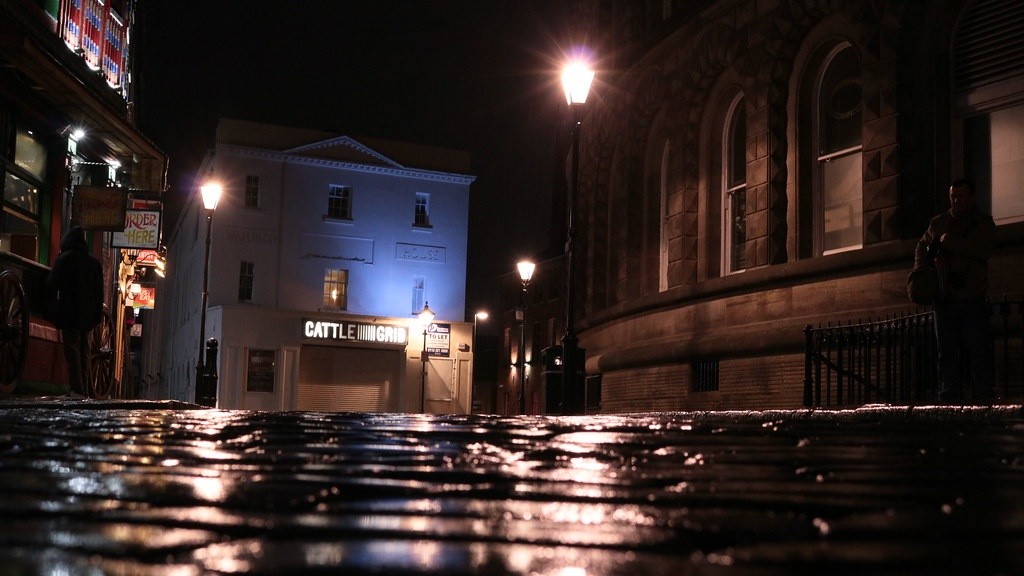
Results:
[545,344,587,415]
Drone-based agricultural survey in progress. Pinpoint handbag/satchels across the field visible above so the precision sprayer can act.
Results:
[906,213,947,306]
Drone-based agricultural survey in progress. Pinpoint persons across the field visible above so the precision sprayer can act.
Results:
[912,177,1001,405]
[43,226,105,401]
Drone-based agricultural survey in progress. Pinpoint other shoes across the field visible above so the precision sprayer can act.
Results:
[59,391,83,400]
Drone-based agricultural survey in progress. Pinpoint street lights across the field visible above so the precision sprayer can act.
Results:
[542,54,598,414]
[473,310,491,352]
[518,256,535,416]
[192,166,224,404]
